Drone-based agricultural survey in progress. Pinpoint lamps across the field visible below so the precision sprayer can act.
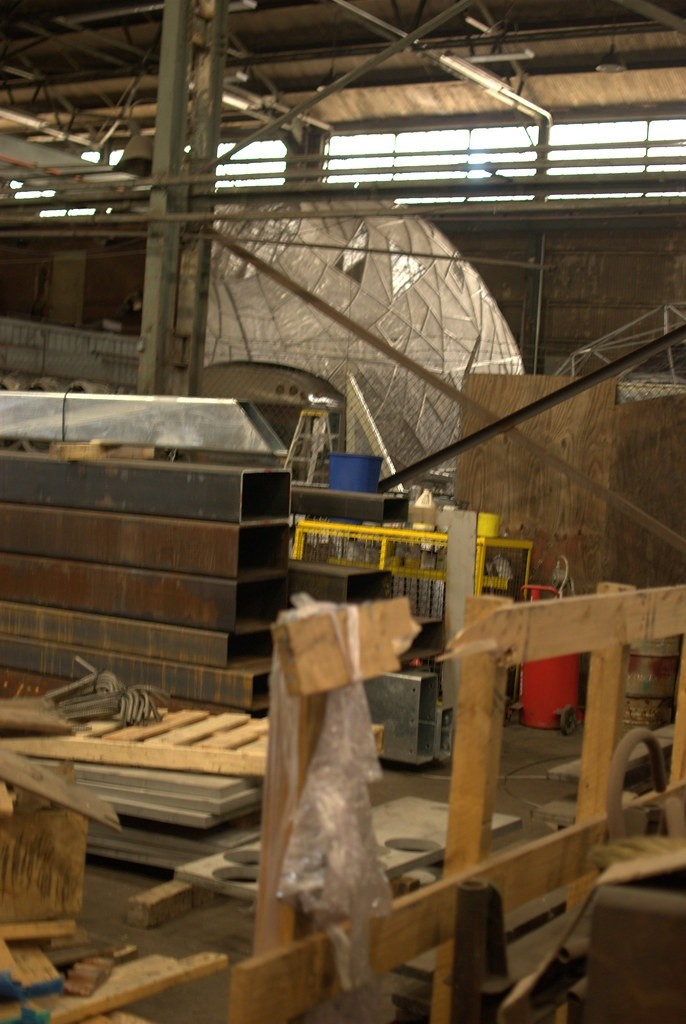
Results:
[595,6,628,74]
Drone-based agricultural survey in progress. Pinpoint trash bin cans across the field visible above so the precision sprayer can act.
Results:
[329,453,382,525]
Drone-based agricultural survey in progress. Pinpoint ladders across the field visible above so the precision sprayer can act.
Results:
[283,409,335,486]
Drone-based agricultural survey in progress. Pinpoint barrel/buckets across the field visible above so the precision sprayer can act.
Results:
[326,451,384,524]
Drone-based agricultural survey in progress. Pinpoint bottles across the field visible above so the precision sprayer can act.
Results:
[411,489,436,532]
